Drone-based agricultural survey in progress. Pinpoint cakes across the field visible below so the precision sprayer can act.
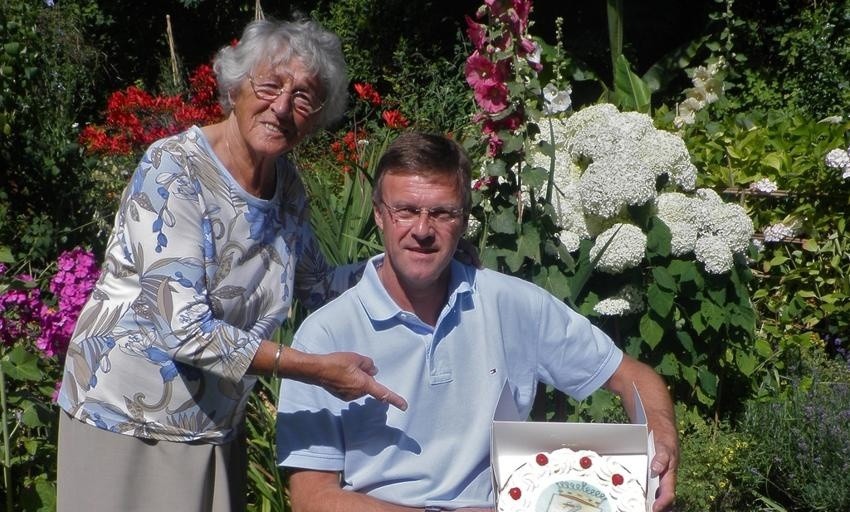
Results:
[498,448,647,512]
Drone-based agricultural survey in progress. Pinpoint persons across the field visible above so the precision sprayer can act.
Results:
[275,131,679,512]
[55,19,409,512]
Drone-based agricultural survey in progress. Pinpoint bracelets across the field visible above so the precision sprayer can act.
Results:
[272,343,283,381]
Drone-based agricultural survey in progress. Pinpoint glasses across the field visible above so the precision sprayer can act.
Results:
[379,197,463,226]
[248,72,331,118]
